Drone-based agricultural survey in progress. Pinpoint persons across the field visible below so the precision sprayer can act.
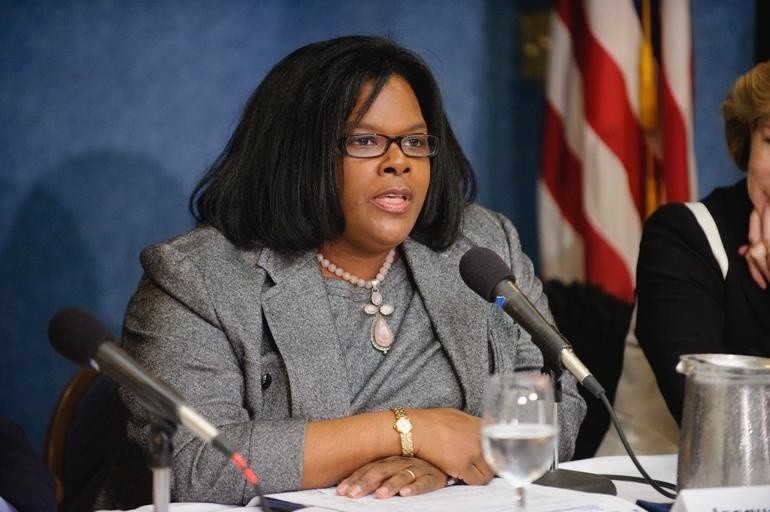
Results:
[118,36,587,512]
[635,60,770,428]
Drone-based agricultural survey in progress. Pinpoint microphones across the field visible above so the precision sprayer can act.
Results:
[459,248,606,399]
[48,308,237,458]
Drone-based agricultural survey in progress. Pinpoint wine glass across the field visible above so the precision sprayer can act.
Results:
[480,373,559,511]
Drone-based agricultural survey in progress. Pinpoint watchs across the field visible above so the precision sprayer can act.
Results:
[391,407,414,457]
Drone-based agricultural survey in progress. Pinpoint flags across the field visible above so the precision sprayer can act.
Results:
[535,0,699,307]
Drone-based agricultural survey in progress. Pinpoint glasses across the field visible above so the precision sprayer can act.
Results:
[337,133,441,159]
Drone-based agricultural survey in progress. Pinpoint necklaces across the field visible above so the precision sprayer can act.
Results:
[313,247,396,355]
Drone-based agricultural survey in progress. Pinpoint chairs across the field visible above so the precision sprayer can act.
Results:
[42,339,154,510]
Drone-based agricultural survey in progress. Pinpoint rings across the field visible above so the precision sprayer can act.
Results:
[405,469,416,482]
